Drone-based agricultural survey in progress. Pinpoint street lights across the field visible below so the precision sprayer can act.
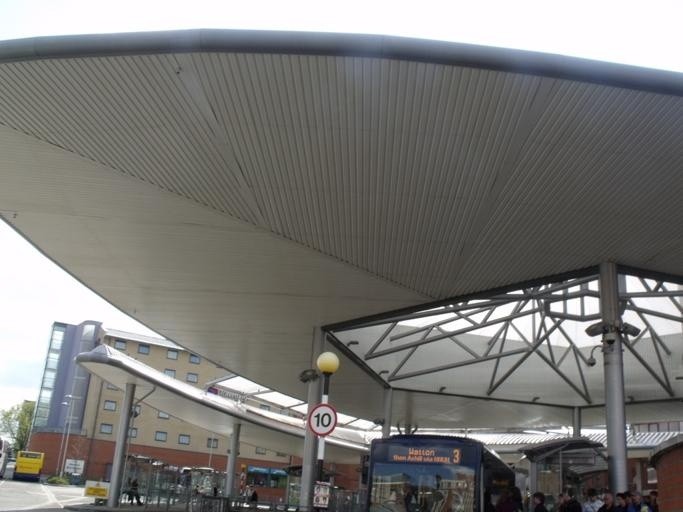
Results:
[55,390,75,480]
[313,347,341,510]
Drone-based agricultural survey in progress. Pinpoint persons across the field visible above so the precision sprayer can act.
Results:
[121,476,134,496]
[127,478,143,506]
[495,484,657,511]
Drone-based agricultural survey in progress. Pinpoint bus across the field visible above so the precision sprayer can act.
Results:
[356,435,520,511]
[122,452,329,511]
[0,438,12,479]
[12,449,45,482]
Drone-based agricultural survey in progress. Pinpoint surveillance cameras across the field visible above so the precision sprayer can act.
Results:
[585,322,607,337]
[587,358,596,367]
[622,322,640,337]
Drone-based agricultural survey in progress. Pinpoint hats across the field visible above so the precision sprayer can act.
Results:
[587,488,600,498]
[630,491,642,496]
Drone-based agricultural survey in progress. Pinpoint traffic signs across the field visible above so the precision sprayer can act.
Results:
[307,403,336,438]
